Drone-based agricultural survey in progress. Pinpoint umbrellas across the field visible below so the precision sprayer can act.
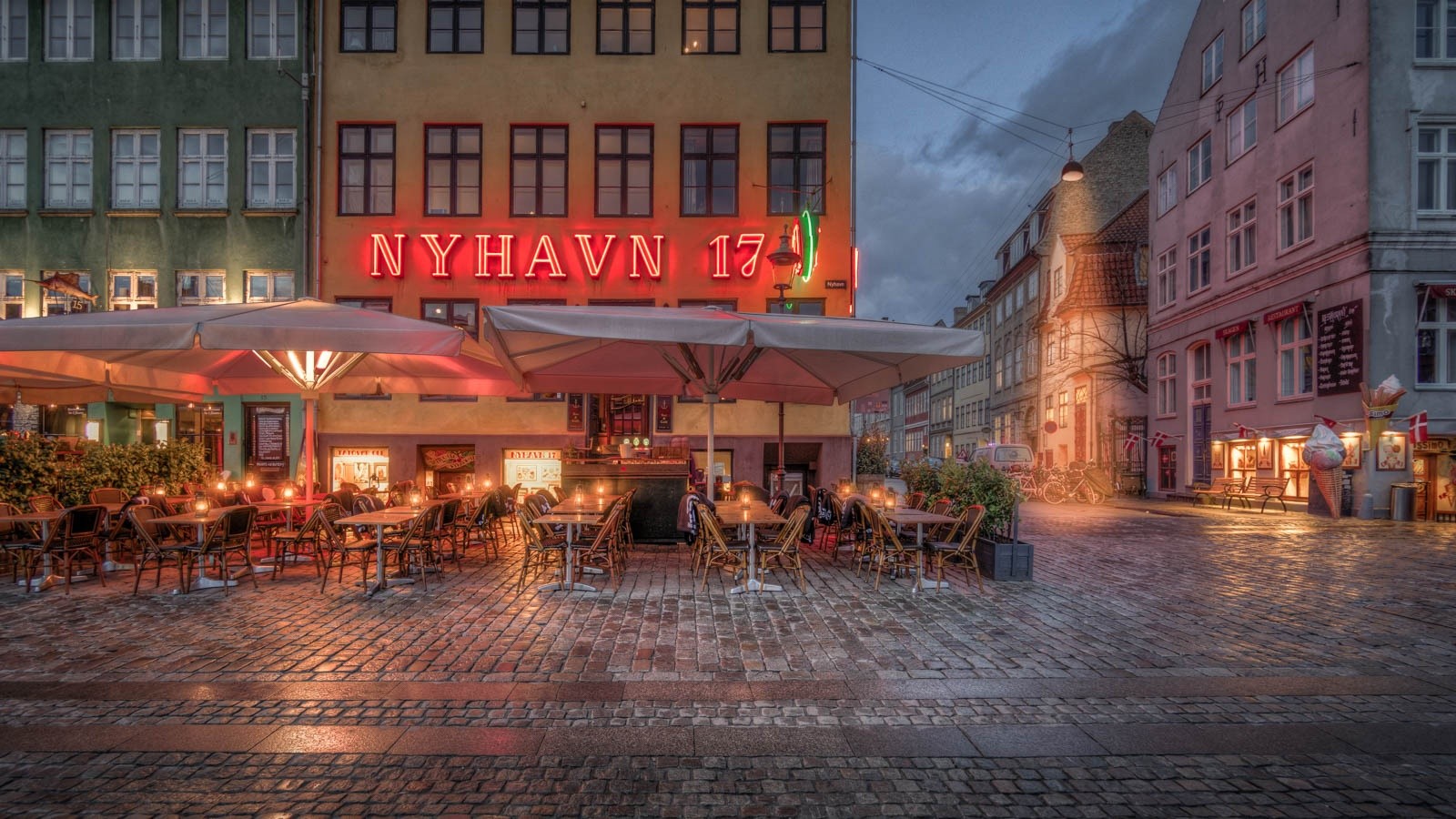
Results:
[0,295,524,558]
[480,303,988,565]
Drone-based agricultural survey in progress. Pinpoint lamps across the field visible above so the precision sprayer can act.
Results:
[1058,128,1085,182]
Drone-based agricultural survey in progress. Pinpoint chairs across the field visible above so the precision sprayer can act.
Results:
[0,471,989,583]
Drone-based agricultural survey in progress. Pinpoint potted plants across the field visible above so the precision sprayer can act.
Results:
[902,459,1033,577]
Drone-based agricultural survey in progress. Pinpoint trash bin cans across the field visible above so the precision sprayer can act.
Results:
[1389,483,1418,521]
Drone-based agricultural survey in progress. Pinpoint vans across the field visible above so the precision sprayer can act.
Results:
[966,443,1036,473]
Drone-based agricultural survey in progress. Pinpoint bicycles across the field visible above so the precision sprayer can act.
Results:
[1005,459,1106,506]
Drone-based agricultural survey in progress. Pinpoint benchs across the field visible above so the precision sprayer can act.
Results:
[1192,476,1247,509]
[1227,476,1291,513]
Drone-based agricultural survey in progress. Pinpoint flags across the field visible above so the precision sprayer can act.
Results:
[1149,432,1169,446]
[1123,434,1140,451]
[1409,411,1429,445]
[1234,421,1251,440]
[1313,414,1338,429]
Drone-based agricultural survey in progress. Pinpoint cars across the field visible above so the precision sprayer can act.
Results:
[884,455,947,479]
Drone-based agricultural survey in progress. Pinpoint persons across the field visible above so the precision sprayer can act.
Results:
[354,462,368,483]
[334,463,343,481]
